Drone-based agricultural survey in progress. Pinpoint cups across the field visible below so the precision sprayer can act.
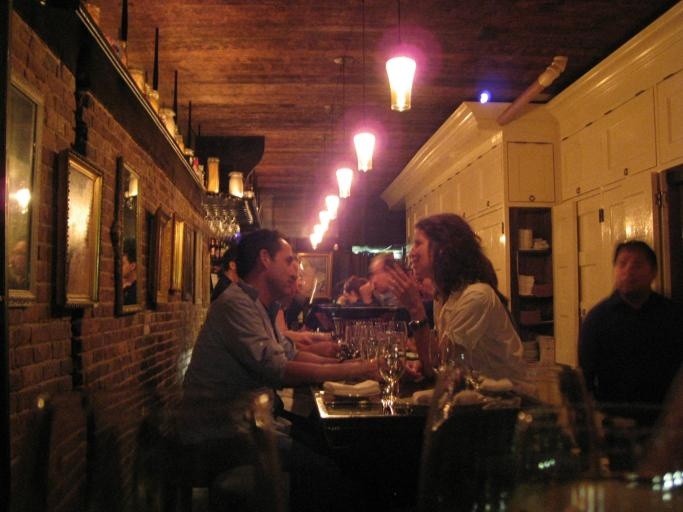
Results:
[507,400,683,512]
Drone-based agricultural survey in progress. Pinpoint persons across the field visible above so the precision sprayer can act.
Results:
[337,254,433,327]
[212,249,339,357]
[6,233,29,290]
[580,232,682,481]
[175,227,377,512]
[120,236,140,308]
[385,213,526,512]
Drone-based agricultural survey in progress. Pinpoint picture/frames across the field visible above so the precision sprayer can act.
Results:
[6,79,45,307]
[57,148,106,313]
[115,150,208,318]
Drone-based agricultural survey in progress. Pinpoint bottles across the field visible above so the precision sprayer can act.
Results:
[203,209,242,265]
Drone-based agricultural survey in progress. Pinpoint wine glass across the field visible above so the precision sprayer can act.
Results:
[334,318,473,408]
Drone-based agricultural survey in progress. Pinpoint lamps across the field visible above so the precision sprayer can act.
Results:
[305,0,416,255]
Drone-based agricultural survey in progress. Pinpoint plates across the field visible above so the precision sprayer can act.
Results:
[517,226,556,363]
[322,402,384,415]
[405,390,493,408]
[318,380,386,404]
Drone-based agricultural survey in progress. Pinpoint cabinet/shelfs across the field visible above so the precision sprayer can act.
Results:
[379,0,682,383]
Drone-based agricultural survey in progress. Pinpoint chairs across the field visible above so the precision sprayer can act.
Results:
[136,333,595,512]
